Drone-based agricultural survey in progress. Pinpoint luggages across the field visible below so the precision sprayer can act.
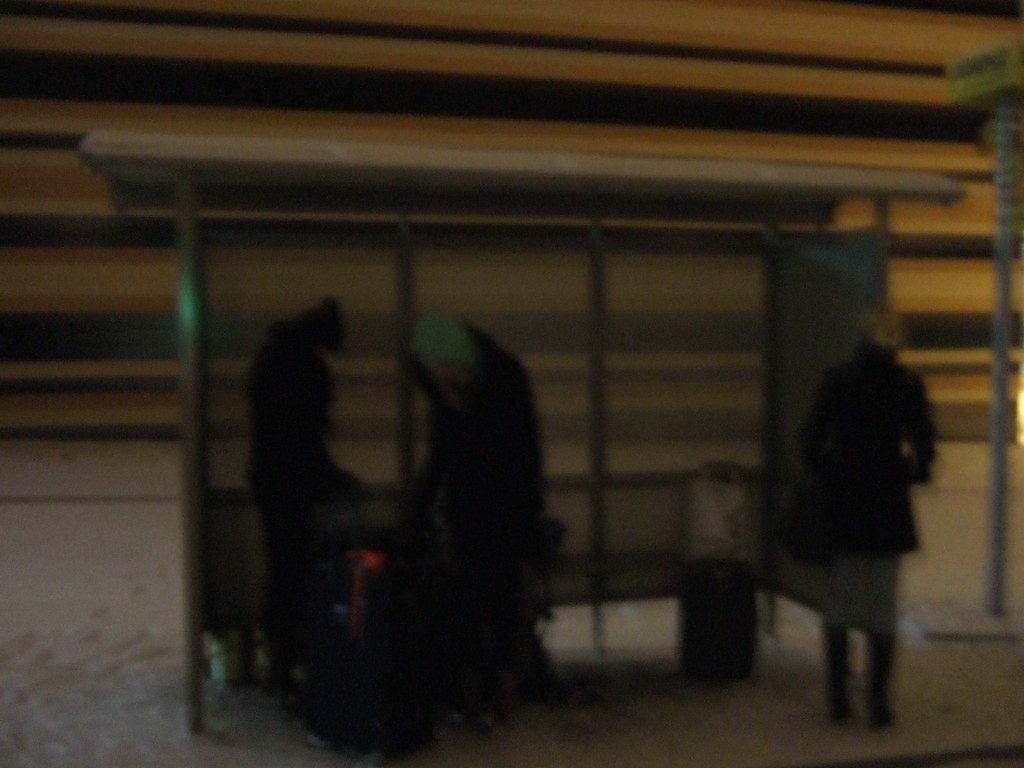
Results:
[305,486,437,761]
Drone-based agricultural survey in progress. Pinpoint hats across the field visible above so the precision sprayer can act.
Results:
[290,299,347,352]
[411,308,471,360]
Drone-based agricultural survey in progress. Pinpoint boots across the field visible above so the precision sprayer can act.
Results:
[869,631,894,727]
[462,666,516,731]
[826,623,856,723]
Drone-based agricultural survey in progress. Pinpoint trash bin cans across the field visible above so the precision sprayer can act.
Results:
[679,560,758,680]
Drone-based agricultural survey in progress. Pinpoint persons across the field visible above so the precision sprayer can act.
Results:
[404,326,601,719]
[247,296,365,704]
[779,310,935,728]
